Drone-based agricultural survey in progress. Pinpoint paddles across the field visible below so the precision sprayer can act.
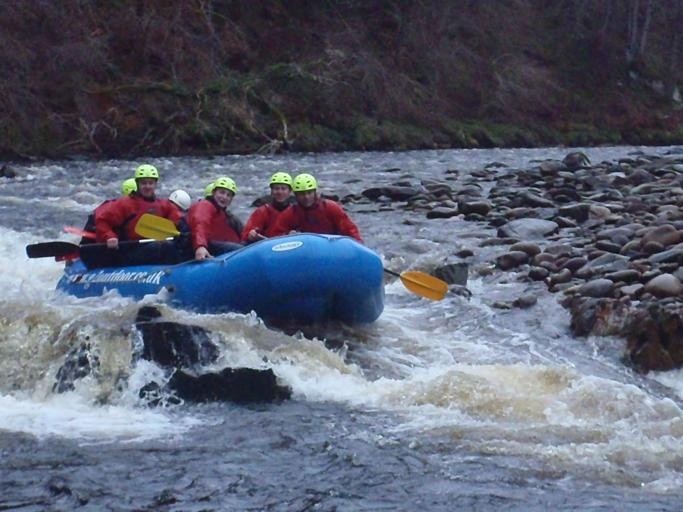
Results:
[63,226,98,239]
[135,213,192,239]
[26,237,177,259]
[383,267,448,302]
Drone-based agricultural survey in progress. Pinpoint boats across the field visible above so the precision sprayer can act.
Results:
[56,233,385,341]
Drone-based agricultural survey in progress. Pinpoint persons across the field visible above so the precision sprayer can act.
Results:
[94,162,189,251]
[77,177,140,244]
[268,172,365,246]
[201,182,214,201]
[172,175,245,263]
[167,188,193,221]
[239,171,295,245]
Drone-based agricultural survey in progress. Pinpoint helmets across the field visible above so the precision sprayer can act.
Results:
[122,178,137,195]
[202,183,213,198]
[134,164,158,190]
[291,173,316,193]
[211,177,236,196]
[168,190,191,212]
[268,172,292,190]
